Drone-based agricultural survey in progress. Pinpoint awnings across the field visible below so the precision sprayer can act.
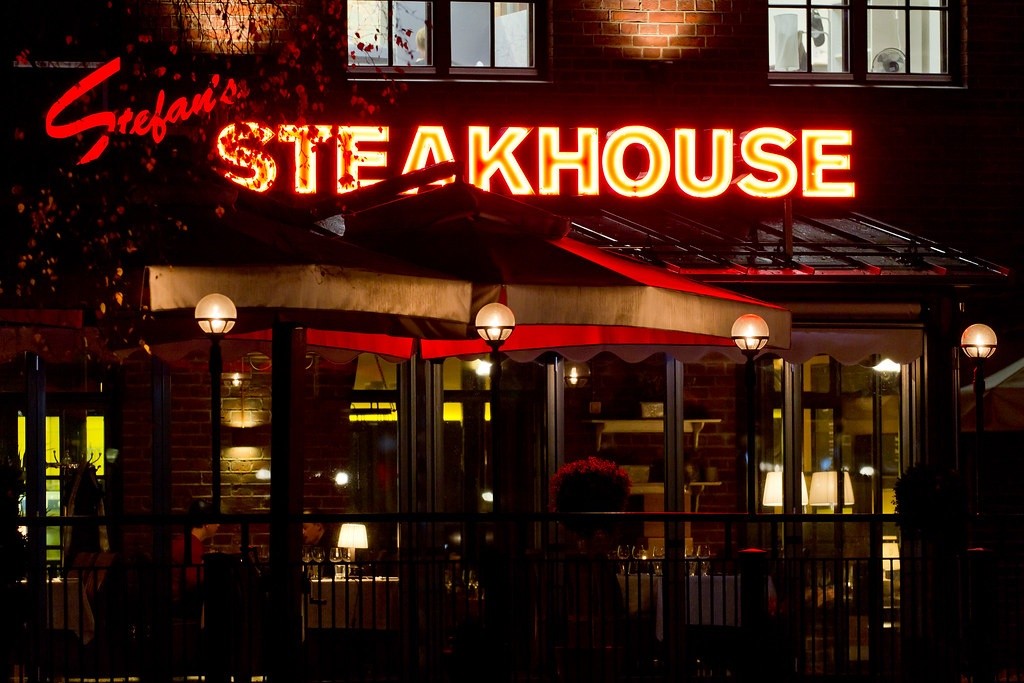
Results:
[544,193,1012,367]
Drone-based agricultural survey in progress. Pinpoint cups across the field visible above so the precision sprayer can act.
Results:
[301,546,314,562]
[312,547,325,562]
[329,548,342,562]
[343,548,354,562]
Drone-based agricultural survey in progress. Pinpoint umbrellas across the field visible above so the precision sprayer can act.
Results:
[0,172,790,683]
[953,357,1024,433]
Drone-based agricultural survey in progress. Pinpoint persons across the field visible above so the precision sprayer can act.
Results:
[525,454,639,683]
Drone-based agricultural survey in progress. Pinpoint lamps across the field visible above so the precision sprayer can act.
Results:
[469,301,516,514]
[193,293,237,511]
[730,315,770,514]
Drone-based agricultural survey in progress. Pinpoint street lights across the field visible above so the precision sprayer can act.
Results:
[474,302,518,512]
[730,312,770,514]
[959,321,999,513]
[195,291,241,519]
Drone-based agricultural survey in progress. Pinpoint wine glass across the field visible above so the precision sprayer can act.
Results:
[617,545,630,575]
[653,545,664,576]
[698,545,710,576]
[685,545,697,576]
[632,545,644,576]
